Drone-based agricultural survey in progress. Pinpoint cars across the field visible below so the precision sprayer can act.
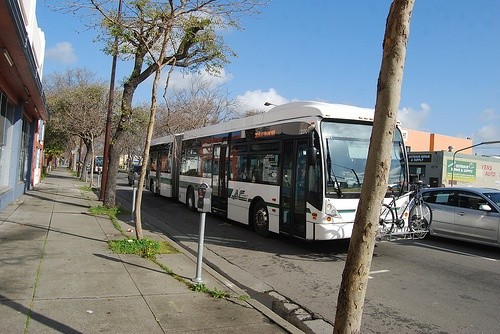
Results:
[408,187,500,249]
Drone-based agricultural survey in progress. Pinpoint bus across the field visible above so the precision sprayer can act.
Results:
[89,156,108,173]
[144,102,414,245]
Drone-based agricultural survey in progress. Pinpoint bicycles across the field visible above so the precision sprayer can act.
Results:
[373,178,432,242]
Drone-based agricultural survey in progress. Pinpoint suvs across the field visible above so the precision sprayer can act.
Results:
[128,160,145,187]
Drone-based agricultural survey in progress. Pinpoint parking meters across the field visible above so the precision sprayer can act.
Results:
[192,182,212,283]
